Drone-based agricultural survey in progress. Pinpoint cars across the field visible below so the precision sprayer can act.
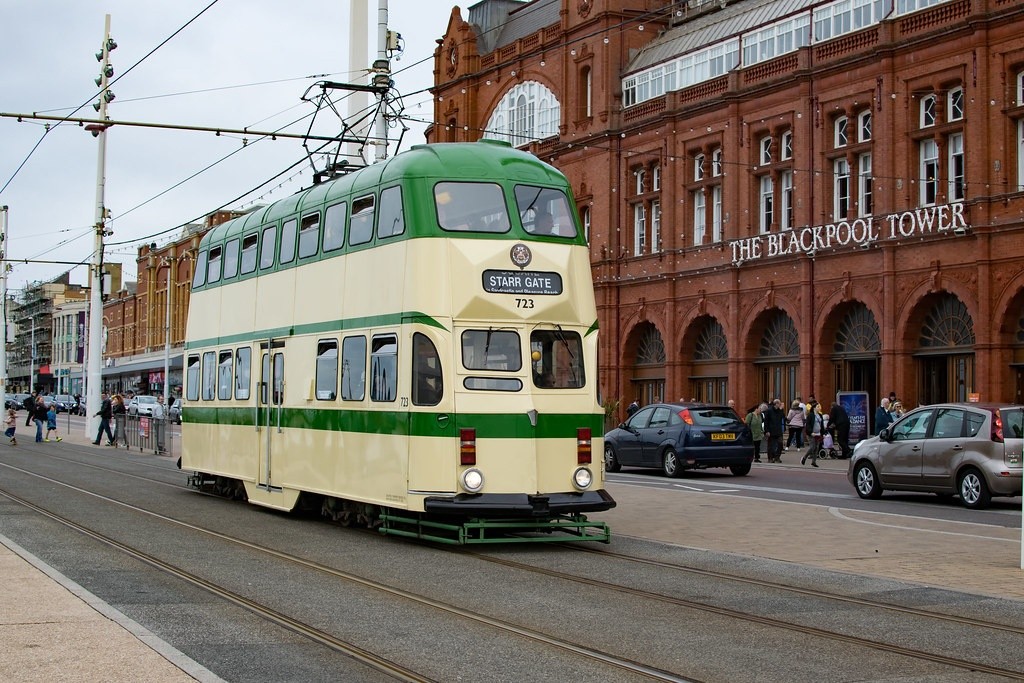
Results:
[4,390,32,411]
[34,395,56,411]
[604,402,756,477]
[79,396,87,417]
[124,394,158,420]
[170,399,182,425]
[51,395,79,415]
[848,403,1024,512]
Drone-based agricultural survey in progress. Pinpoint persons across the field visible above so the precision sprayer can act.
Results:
[40,390,45,395]
[168,394,175,409]
[23,389,29,393]
[92,392,113,445]
[44,405,62,442]
[3,409,17,445]
[32,396,47,442]
[64,391,68,395]
[418,357,440,400]
[541,373,556,387]
[152,394,166,452]
[531,212,556,234]
[653,396,663,404]
[728,400,735,409]
[942,417,955,437]
[24,392,36,426]
[680,397,696,402]
[626,398,639,418]
[745,395,826,467]
[917,400,925,406]
[75,394,82,404]
[875,392,905,436]
[827,403,851,459]
[106,395,127,447]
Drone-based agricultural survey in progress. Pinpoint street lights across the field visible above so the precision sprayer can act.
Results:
[28,316,34,394]
[84,123,115,446]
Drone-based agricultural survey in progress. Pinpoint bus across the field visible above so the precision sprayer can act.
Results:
[177,137,618,547]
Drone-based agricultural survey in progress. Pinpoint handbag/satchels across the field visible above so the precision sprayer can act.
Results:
[822,432,834,448]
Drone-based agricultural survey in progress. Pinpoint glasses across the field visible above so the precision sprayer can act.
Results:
[895,405,901,408]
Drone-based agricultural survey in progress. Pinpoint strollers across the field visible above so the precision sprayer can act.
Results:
[815,425,839,461]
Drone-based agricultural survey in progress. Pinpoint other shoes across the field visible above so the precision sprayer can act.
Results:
[11,442,17,445]
[838,456,847,459]
[774,457,782,463]
[26,424,31,426]
[801,457,805,465]
[36,439,44,442]
[797,448,801,451]
[785,446,789,450]
[56,438,62,442]
[105,443,113,446]
[92,442,100,445]
[754,459,761,463]
[811,462,819,467]
[44,439,50,442]
[158,446,166,451]
[10,437,15,442]
[768,458,774,463]
